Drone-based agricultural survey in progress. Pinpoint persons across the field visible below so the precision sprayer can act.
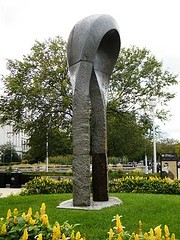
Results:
[157,163,161,173]
[148,164,152,174]
[132,161,137,167]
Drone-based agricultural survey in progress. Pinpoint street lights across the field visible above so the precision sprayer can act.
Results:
[148,96,158,173]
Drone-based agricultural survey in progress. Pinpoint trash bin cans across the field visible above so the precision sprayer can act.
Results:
[9,172,22,188]
[0,173,7,187]
